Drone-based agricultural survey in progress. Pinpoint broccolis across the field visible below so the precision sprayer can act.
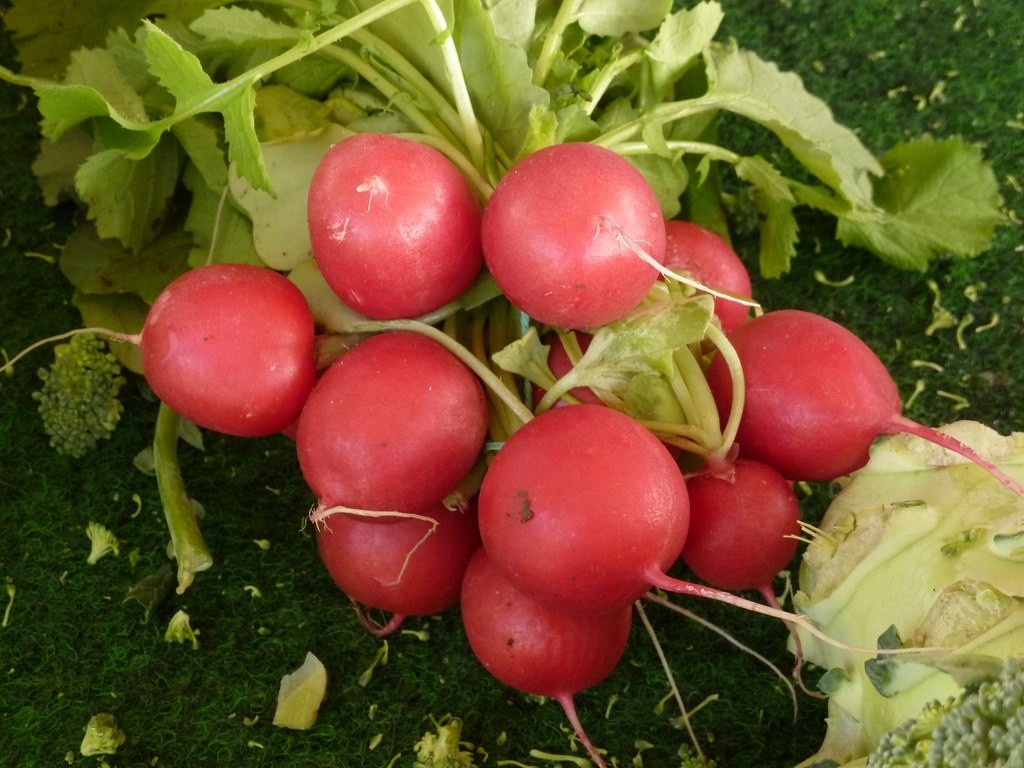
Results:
[31,331,126,457]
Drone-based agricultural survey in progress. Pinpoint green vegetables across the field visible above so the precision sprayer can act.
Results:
[863,652,1024,768]
